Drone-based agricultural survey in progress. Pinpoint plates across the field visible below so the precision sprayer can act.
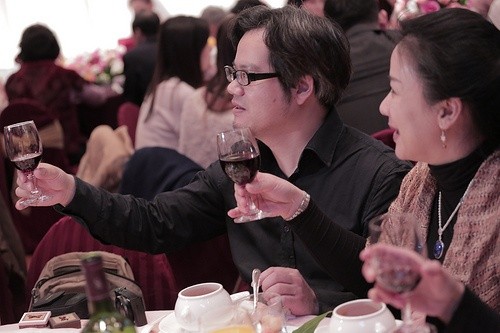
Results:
[141,309,175,333]
[314,316,331,333]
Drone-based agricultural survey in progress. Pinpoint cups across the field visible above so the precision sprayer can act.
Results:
[328,299,397,333]
[234,292,288,333]
[175,282,233,330]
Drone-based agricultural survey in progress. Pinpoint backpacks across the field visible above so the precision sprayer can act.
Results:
[28,251,146,319]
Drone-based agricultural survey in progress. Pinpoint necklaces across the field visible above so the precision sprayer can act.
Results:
[433,191,460,259]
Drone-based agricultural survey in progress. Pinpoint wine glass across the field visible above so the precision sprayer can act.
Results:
[4,120,51,206]
[216,127,272,223]
[368,212,432,333]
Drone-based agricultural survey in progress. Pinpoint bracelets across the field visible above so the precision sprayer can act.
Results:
[285,190,311,222]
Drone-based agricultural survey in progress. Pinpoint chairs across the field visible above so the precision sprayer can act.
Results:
[0,98,395,315]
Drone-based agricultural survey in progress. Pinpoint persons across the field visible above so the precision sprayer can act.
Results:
[359,243,500,333]
[5,0,415,320]
[227,8,500,333]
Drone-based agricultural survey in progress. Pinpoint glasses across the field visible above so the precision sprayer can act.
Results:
[223,65,278,86]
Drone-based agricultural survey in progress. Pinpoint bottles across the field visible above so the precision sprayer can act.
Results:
[78,252,136,333]
